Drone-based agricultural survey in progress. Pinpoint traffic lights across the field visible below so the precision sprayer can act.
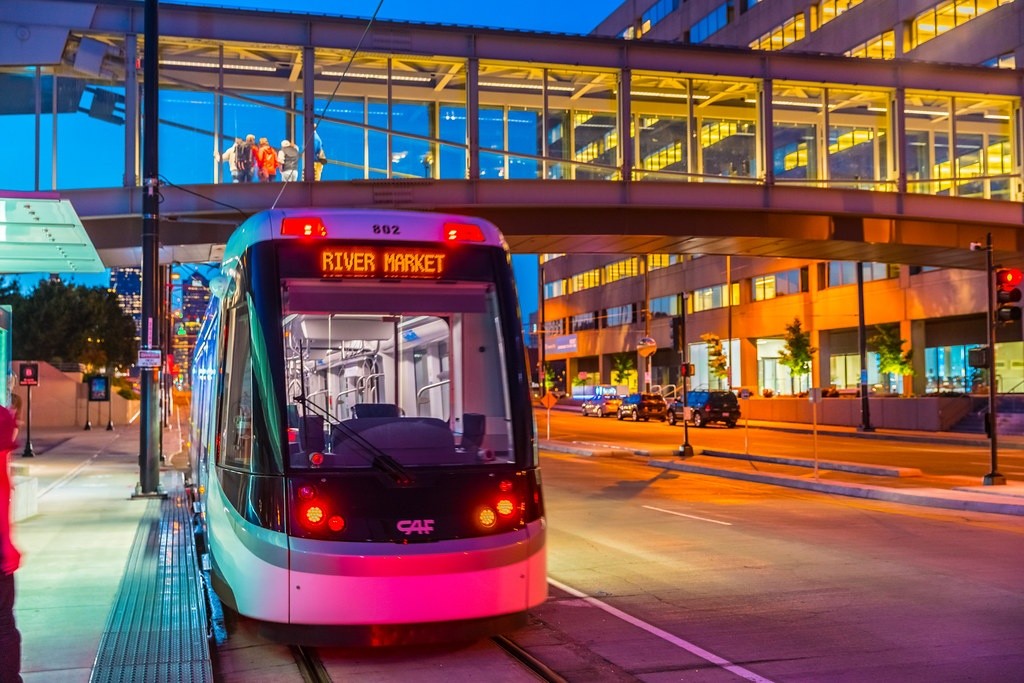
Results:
[991,265,1021,326]
[681,362,695,376]
[669,316,682,354]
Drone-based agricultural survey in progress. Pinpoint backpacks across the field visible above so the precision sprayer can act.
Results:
[235,145,252,172]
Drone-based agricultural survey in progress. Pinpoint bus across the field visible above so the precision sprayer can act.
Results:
[188,208,547,651]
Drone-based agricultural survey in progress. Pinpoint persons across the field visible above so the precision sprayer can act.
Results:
[313,123,327,182]
[0,371,23,683]
[222,137,242,183]
[234,133,257,184]
[422,151,434,179]
[277,140,299,182]
[854,175,861,189]
[256,137,278,183]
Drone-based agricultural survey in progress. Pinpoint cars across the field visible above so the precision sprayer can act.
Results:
[582,394,622,417]
[617,393,667,423]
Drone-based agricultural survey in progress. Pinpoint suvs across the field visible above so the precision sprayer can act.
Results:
[665,388,740,428]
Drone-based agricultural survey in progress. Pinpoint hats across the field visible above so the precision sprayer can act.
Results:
[281,140,290,147]
